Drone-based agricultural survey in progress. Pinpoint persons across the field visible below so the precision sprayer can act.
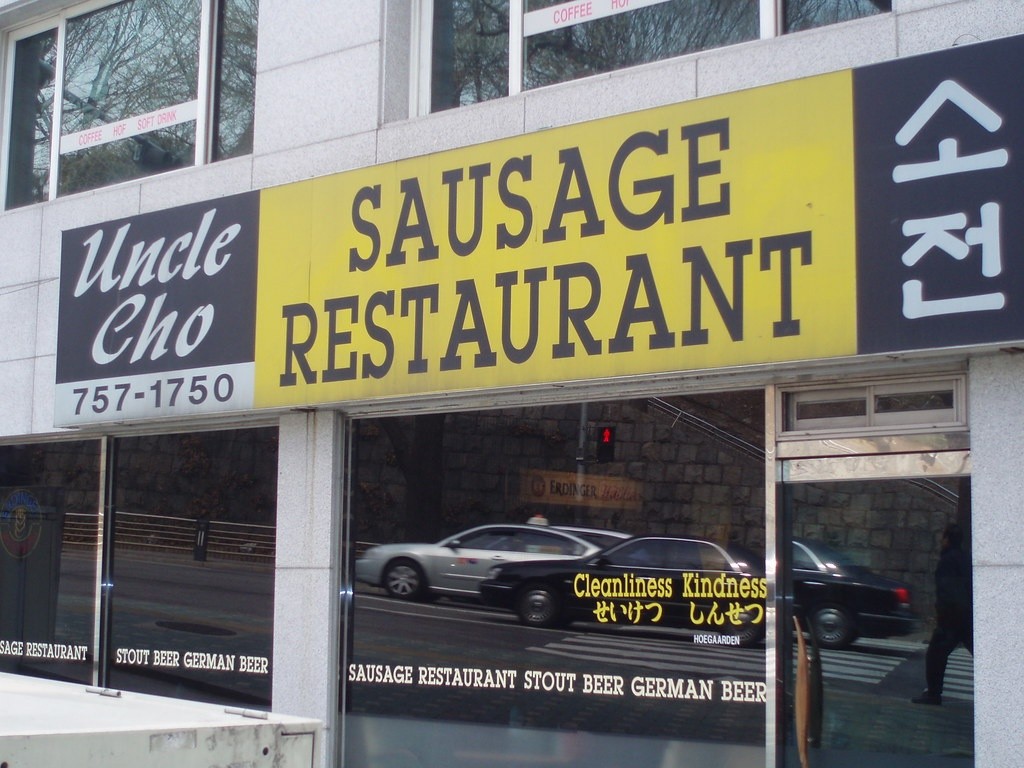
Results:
[911,523,974,704]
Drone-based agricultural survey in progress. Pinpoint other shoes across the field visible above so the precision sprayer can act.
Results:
[912,691,941,704]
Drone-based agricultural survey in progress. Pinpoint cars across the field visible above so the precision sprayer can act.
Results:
[352,522,605,603]
[792,533,924,651]
[476,532,766,646]
[485,509,647,564]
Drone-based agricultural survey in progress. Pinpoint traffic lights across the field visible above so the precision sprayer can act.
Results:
[595,425,617,463]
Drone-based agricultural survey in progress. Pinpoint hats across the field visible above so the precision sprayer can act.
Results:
[628,548,648,560]
